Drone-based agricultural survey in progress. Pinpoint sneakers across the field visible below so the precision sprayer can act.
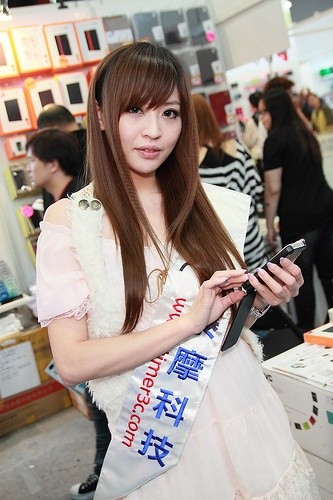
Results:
[70,466,102,500]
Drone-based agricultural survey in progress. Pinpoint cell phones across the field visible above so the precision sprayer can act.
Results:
[241,241,307,293]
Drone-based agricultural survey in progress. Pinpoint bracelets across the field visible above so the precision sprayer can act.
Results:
[250,305,270,318]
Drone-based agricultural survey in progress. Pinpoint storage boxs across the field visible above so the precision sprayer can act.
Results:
[0,324,74,437]
[260,342,333,500]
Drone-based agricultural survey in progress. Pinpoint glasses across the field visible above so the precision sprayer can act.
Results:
[255,107,268,119]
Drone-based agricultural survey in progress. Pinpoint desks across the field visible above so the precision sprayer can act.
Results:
[0,292,31,314]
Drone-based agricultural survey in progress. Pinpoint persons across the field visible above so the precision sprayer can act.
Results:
[244,78,333,183]
[37,103,89,213]
[34,41,321,500]
[26,127,111,500]
[191,95,267,273]
[258,89,333,330]
